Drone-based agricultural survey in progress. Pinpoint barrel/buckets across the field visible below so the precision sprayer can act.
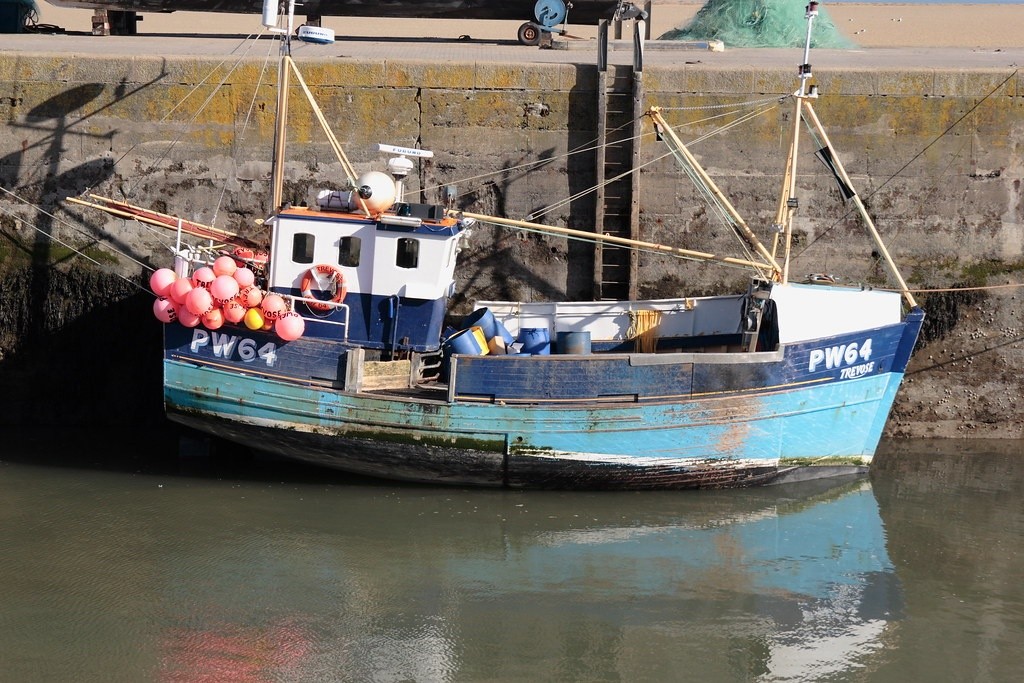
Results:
[462,307,513,348]
[518,328,550,355]
[469,326,490,356]
[447,329,482,356]
[556,331,591,354]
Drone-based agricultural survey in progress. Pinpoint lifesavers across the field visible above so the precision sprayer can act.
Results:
[300,265,347,311]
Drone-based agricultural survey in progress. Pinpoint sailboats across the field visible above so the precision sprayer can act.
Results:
[64,1,928,493]
